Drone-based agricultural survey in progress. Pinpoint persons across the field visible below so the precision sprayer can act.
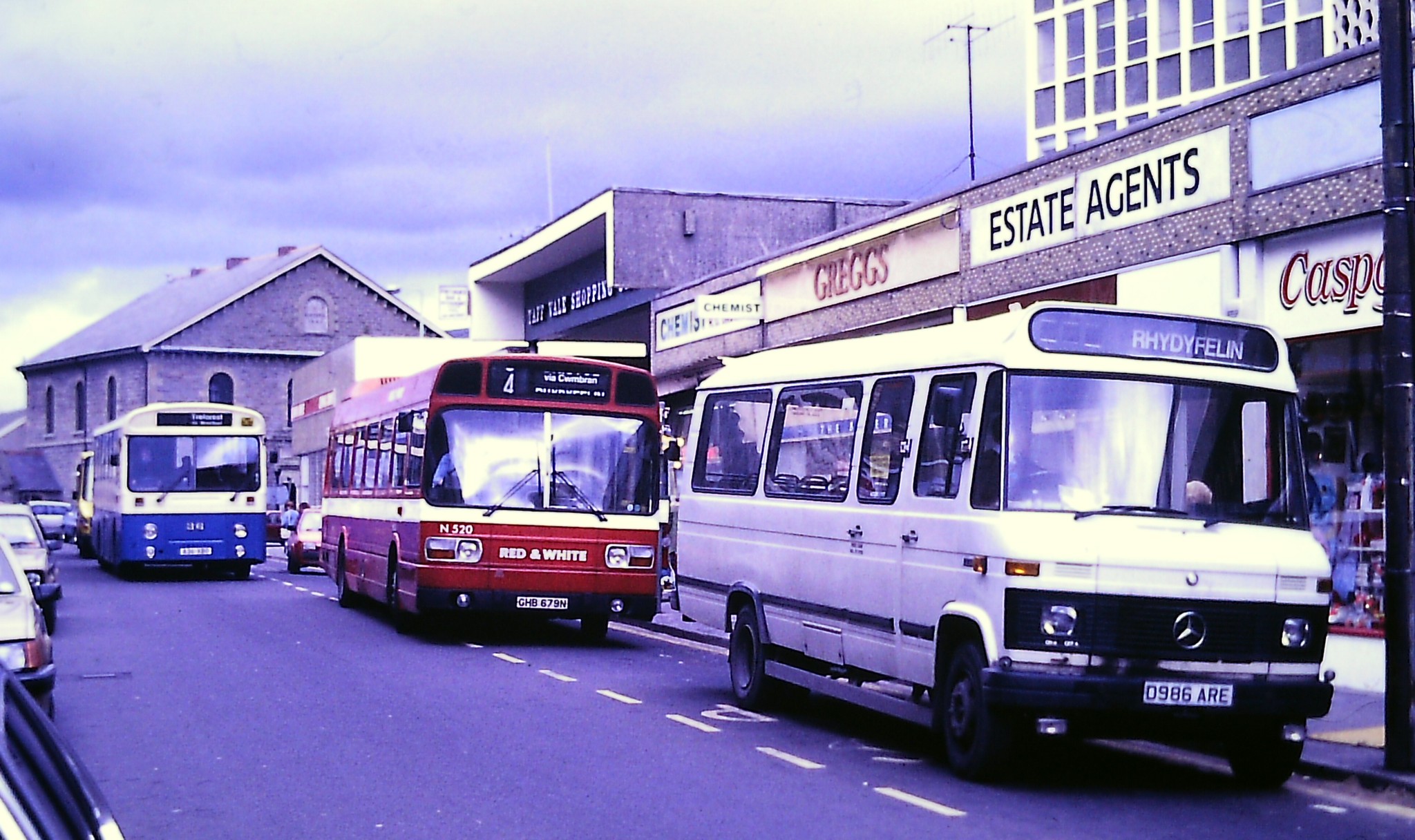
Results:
[131,445,164,486]
[431,454,462,490]
[280,502,299,531]
[299,502,310,514]
[974,411,1059,511]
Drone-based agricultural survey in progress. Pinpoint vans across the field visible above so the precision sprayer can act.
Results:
[27,501,80,550]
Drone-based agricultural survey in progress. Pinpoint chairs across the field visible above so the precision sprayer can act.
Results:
[716,473,847,494]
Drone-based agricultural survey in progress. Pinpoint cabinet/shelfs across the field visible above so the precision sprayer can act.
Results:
[1340,509,1385,623]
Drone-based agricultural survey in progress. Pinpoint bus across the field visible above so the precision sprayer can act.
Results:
[75,402,279,581]
[322,356,664,642]
[675,300,1337,789]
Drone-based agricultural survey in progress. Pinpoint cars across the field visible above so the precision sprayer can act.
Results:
[0,502,63,723]
[268,512,289,546]
[284,506,329,573]
